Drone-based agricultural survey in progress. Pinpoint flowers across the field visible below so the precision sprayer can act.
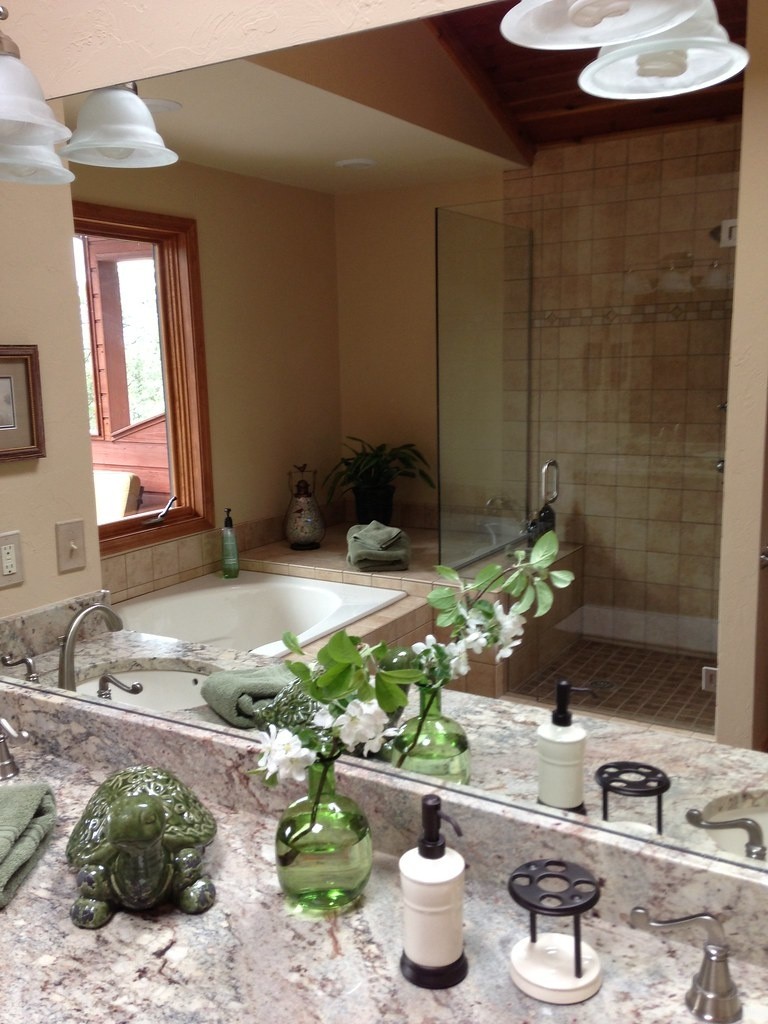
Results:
[339,531,576,771]
[246,626,454,868]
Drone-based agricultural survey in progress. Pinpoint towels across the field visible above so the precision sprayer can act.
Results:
[353,520,402,550]
[346,523,411,572]
[200,666,293,726]
[0,784,59,908]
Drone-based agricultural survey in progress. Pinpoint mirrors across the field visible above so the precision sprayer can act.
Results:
[0,1,768,872]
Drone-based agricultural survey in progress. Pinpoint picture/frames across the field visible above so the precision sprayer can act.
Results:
[1,343,47,465]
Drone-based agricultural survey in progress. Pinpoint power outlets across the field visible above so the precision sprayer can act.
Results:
[0,532,26,591]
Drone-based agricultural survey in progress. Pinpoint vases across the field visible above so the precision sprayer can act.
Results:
[389,679,472,786]
[273,761,371,915]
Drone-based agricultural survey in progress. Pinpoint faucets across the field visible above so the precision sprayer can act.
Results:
[59,604,123,691]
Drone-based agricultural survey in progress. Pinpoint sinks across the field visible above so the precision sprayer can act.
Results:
[66,656,234,711]
[702,788,767,861]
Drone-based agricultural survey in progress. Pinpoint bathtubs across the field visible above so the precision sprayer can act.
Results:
[110,567,407,662]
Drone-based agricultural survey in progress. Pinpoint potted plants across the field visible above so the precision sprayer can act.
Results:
[321,433,435,525]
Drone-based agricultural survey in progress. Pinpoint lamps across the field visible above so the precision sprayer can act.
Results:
[501,1,751,101]
[63,82,179,171]
[0,8,76,186]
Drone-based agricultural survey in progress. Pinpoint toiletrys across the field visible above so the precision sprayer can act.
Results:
[399,794,470,990]
[536,681,598,817]
[221,508,240,578]
